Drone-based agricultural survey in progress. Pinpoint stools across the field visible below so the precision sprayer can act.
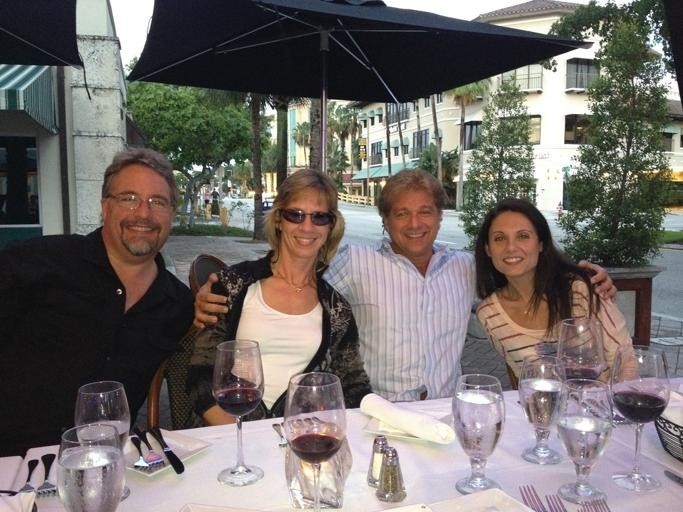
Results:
[602,279,650,349]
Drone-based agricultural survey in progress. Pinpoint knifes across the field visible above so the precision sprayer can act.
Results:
[664,470,683,487]
[151,427,185,477]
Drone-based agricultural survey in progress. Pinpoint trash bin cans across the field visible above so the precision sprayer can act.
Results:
[231,193,236,198]
[261,201,273,216]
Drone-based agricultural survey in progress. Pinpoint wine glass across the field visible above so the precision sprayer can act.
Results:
[210,338,269,488]
[286,372,347,510]
[553,317,604,385]
[453,344,670,505]
[54,377,131,512]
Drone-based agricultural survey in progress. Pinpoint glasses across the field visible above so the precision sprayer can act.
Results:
[277,208,336,226]
[108,192,175,211]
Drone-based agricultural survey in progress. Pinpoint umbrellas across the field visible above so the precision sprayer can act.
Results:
[123,0,599,182]
[0,1,85,73]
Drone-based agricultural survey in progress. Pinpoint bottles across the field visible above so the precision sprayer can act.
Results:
[367,435,406,505]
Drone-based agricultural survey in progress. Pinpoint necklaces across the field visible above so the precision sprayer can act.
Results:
[273,269,315,295]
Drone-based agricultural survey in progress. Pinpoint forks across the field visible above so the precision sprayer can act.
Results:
[519,484,613,512]
[129,426,165,474]
[270,422,289,451]
[18,453,58,502]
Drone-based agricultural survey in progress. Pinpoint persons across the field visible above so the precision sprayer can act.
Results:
[192,166,619,408]
[204,190,210,203]
[181,165,378,429]
[472,195,643,389]
[210,186,220,202]
[1,147,204,459]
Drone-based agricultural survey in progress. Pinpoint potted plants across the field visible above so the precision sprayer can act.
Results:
[540,1,683,280]
